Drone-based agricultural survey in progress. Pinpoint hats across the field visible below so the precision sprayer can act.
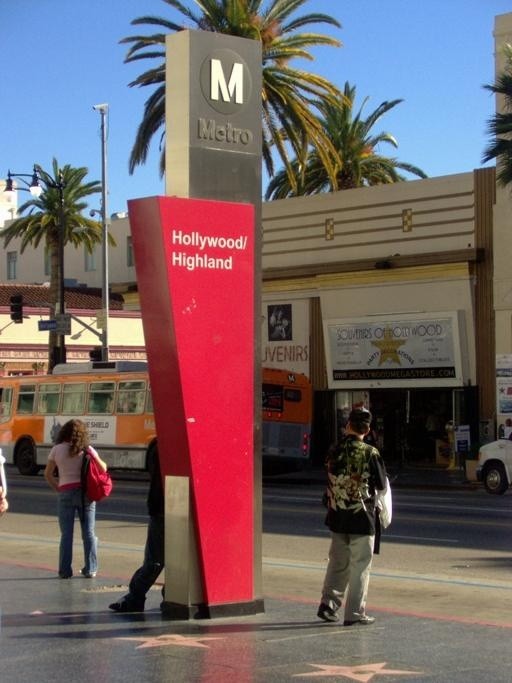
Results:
[350,408,372,423]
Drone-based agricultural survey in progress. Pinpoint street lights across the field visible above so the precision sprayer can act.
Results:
[4,168,67,362]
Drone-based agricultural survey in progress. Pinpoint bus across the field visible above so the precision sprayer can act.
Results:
[0,361,314,480]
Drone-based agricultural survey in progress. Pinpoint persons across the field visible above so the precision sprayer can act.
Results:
[44,419,108,578]
[0,448,8,521]
[269,306,288,340]
[108,437,165,612]
[316,406,387,625]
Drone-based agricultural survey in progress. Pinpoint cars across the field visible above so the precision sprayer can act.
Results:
[476,437,512,494]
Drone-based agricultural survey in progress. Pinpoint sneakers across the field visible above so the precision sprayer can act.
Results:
[318,610,339,622]
[109,602,144,612]
[344,615,376,626]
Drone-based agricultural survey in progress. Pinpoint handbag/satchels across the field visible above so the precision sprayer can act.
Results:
[81,447,113,502]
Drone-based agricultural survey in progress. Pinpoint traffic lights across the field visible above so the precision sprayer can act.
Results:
[10,292,24,321]
